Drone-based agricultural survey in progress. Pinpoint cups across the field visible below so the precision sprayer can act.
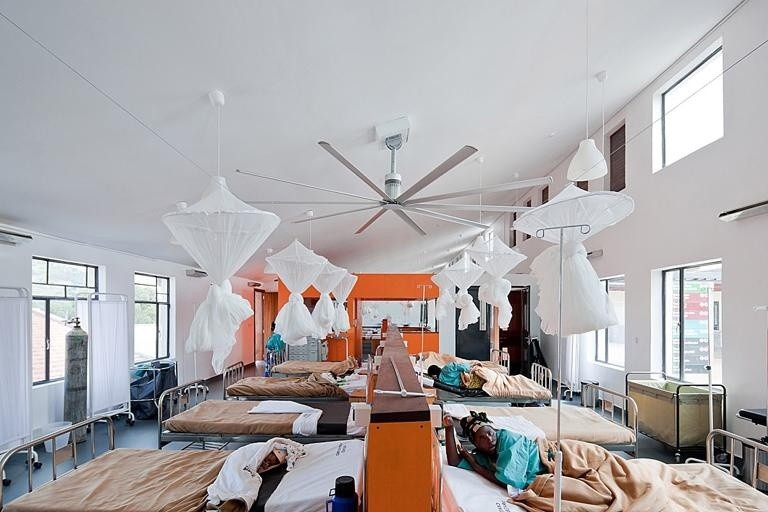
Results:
[329,475,356,498]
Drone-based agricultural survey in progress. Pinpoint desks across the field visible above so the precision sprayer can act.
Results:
[738,407,767,493]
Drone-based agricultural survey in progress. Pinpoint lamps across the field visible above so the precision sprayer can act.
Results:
[566,0,608,183]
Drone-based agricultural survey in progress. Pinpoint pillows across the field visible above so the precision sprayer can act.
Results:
[265,438,365,511]
[436,461,527,511]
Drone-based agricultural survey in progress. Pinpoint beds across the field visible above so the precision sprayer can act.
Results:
[222,360,373,403]
[410,348,510,373]
[417,361,552,407]
[439,428,767,512]
[269,350,375,376]
[0,415,368,511]
[157,377,370,450]
[430,383,640,459]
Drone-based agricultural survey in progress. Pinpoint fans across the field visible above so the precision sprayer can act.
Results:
[235,134,554,236]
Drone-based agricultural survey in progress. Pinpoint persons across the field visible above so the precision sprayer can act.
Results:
[443,411,549,490]
[265,321,285,377]
[262,448,287,469]
[428,362,486,390]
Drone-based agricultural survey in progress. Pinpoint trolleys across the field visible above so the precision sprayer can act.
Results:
[130,361,178,415]
